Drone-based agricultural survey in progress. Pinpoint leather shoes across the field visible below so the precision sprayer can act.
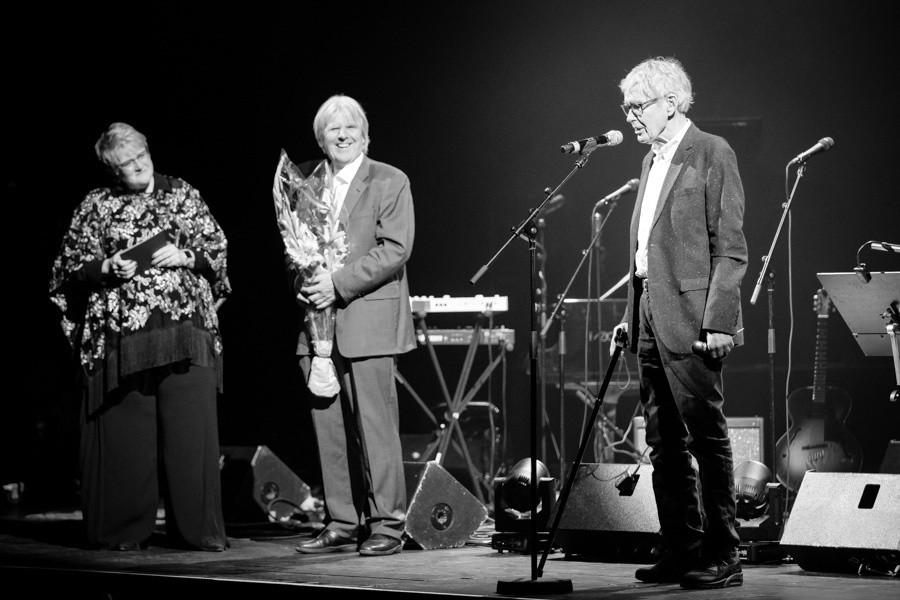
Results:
[359,532,402,556]
[294,529,358,553]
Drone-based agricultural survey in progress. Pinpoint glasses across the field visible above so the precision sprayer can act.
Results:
[621,95,667,116]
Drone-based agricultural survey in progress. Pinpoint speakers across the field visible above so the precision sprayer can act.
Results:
[404,461,486,550]
[782,470,900,575]
[548,461,664,558]
[215,445,312,534]
[632,414,764,471]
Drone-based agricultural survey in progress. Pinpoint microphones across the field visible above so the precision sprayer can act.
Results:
[597,179,640,207]
[561,129,624,153]
[789,137,834,166]
[615,465,639,488]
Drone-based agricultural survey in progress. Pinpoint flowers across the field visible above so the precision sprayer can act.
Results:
[271,148,349,399]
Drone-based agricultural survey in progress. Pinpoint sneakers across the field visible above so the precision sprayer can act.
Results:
[679,562,742,588]
[635,560,680,584]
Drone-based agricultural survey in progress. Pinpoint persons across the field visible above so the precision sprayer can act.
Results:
[609,56,749,591]
[46,119,233,557]
[284,94,419,557]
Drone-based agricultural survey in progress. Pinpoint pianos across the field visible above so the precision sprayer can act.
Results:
[409,294,514,517]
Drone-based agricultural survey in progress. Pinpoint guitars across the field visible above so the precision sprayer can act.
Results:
[776,287,863,493]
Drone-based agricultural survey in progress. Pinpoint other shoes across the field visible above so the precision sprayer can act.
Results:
[200,543,223,552]
[114,542,129,552]
